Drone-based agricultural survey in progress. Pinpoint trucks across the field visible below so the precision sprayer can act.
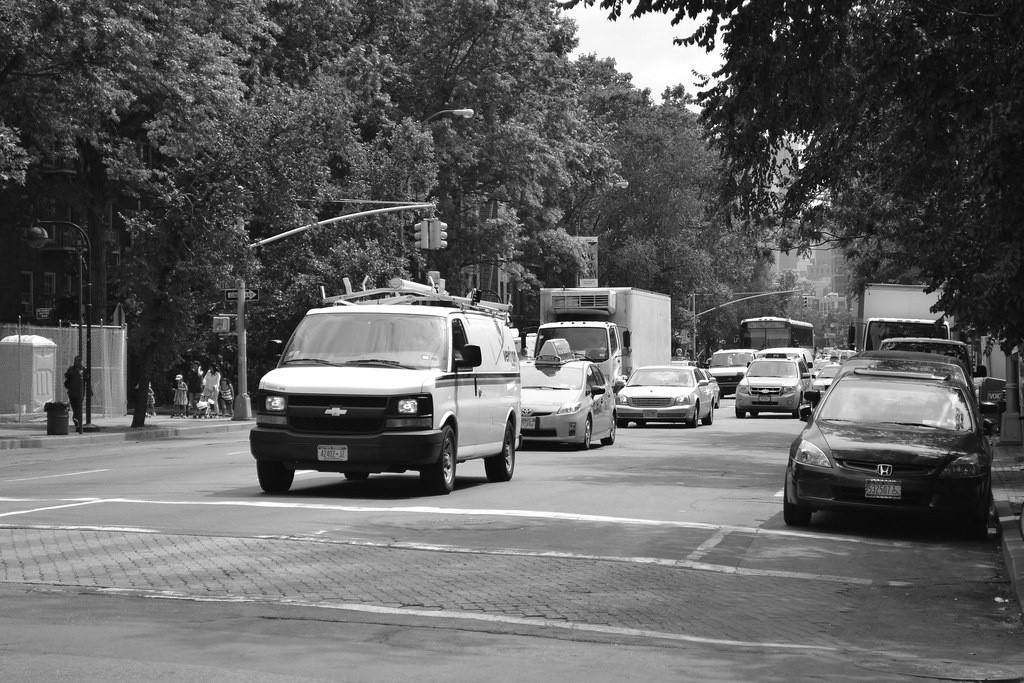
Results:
[846,281,963,354]
[520,285,672,424]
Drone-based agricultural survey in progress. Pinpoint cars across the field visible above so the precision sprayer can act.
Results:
[733,317,1001,421]
[613,359,721,429]
[782,363,1002,541]
[734,356,814,420]
[519,337,618,452]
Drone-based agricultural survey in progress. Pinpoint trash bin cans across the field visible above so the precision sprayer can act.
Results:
[46,403,69,435]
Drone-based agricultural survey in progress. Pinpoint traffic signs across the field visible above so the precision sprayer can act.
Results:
[224,287,260,302]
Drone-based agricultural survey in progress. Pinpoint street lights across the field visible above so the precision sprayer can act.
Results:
[24,218,101,433]
[397,107,475,279]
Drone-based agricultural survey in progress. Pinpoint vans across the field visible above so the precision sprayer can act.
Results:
[247,276,523,497]
[705,347,760,400]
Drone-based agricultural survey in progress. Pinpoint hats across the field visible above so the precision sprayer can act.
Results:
[175,374,183,381]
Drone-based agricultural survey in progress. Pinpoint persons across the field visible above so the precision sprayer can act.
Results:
[173,374,189,419]
[187,361,234,419]
[146,383,157,417]
[64,356,88,427]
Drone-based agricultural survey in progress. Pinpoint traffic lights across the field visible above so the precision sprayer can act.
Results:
[803,296,808,308]
[413,220,430,250]
[431,220,448,250]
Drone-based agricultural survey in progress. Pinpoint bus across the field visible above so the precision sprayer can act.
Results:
[733,315,815,360]
